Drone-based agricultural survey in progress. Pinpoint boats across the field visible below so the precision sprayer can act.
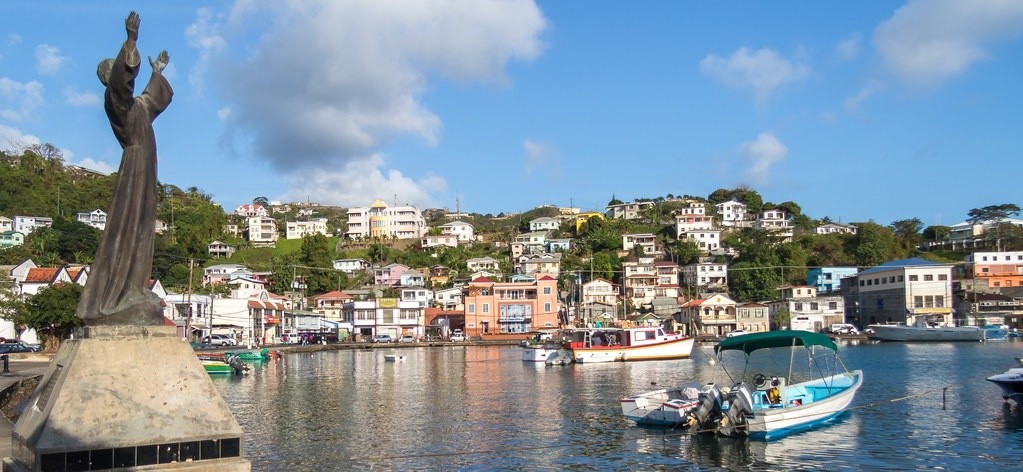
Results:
[196,352,251,374]
[685,330,863,442]
[522,326,695,362]
[867,314,984,342]
[619,383,703,425]
[1014,357,1023,366]
[985,368,1023,406]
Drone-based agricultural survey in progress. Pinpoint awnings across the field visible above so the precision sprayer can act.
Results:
[906,308,956,314]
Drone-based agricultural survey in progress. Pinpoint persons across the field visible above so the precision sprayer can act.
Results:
[254,336,260,349]
[301,334,313,347]
[283,335,287,345]
[848,326,851,333]
[262,335,266,347]
[77,11,174,320]
[271,335,275,345]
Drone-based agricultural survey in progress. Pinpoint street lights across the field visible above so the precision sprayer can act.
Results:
[375,293,378,336]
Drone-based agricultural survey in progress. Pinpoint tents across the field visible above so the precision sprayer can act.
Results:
[713,330,854,409]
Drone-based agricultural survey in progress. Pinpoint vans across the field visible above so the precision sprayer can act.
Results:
[832,324,857,332]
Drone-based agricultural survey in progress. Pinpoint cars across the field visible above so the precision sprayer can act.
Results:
[280,333,298,343]
[202,334,231,346]
[370,335,391,343]
[449,333,469,342]
[865,328,875,334]
[0,343,31,354]
[453,329,463,336]
[835,326,857,335]
[994,324,1009,330]
[558,329,574,337]
[4,339,37,352]
[394,336,415,343]
[218,334,237,346]
[725,329,748,338]
[532,332,552,340]
